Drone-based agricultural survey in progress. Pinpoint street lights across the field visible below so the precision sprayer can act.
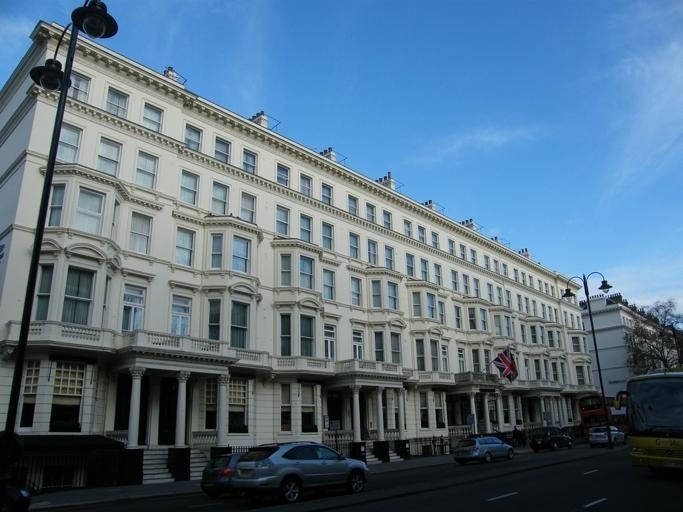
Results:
[561,271,616,449]
[0,1,120,471]
[657,324,682,368]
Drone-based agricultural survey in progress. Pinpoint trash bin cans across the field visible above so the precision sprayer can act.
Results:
[422,445,432,456]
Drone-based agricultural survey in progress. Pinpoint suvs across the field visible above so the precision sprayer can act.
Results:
[232,440,371,502]
[530,426,573,453]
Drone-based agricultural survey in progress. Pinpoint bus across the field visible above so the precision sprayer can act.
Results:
[615,366,683,475]
[578,392,627,444]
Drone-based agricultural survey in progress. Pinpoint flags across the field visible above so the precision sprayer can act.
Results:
[493,350,518,384]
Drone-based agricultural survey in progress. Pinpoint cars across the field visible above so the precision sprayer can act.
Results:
[589,425,625,447]
[0,482,31,512]
[454,437,514,465]
[201,453,248,499]
